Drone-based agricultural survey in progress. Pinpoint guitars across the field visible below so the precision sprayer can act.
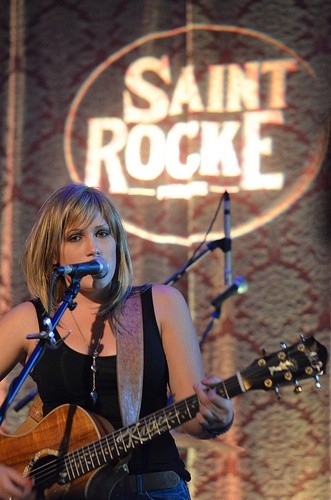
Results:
[0,333,328,500]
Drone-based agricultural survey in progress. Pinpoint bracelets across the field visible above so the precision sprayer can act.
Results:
[208,412,235,435]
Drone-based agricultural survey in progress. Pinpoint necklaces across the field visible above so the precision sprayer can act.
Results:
[68,309,105,403]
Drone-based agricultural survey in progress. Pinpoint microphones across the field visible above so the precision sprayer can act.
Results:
[53,257,110,279]
[223,191,232,286]
[211,277,249,306]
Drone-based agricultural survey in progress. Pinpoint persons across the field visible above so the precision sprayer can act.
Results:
[0,184,235,500]
